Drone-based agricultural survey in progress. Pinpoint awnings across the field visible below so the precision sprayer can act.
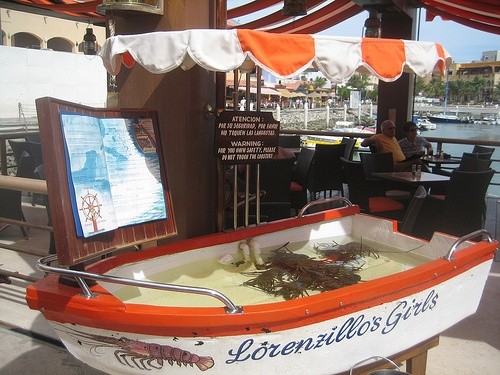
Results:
[225,73,257,89]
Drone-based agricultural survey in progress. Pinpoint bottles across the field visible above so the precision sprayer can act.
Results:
[300,140,307,148]
[412,164,421,176]
[440,151,444,159]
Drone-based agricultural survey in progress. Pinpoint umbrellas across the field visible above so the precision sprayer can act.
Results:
[96,29,453,109]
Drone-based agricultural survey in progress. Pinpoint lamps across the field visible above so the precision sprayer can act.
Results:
[226,86,233,97]
[283,0,306,16]
[364,8,381,38]
[82,20,97,55]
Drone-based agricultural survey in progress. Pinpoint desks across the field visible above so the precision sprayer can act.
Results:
[285,147,302,161]
[420,157,461,170]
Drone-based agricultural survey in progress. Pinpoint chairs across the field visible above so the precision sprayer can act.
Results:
[0,140,49,240]
[267,134,495,242]
[371,172,451,200]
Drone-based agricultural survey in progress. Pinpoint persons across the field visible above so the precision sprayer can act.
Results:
[361,120,431,201]
[241,97,254,111]
[289,98,304,109]
[397,121,433,166]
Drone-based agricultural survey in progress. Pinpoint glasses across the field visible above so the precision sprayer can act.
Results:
[388,126,396,130]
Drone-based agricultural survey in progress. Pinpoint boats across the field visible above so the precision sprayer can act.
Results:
[300,122,376,151]
[25,197,499,375]
[413,112,460,133]
